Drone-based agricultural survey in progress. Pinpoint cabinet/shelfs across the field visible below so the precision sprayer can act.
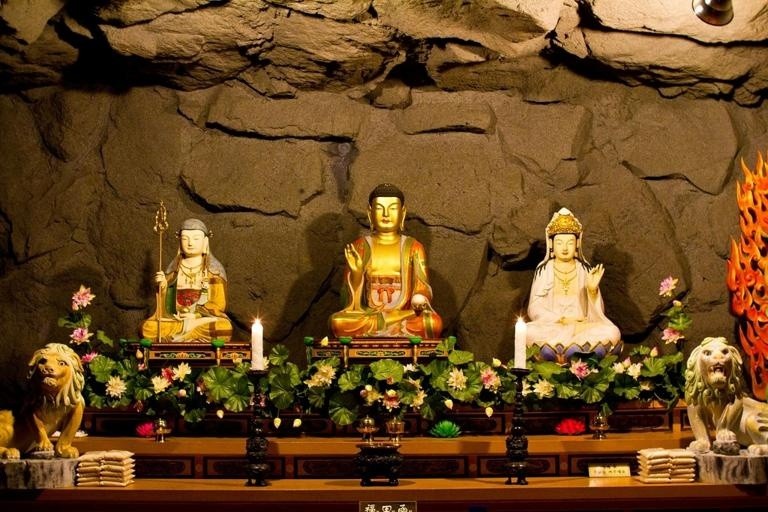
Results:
[0,431,768,512]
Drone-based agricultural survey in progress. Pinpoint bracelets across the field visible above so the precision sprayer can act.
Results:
[588,288,599,297]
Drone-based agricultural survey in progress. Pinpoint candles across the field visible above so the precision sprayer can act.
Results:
[514,317,527,369]
[251,318,265,371]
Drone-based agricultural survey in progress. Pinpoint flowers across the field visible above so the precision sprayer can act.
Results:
[56,275,694,426]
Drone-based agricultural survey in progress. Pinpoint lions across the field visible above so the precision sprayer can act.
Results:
[682,336,767,456]
[0,342,87,461]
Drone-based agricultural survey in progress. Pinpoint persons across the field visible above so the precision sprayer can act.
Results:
[520,206,623,356]
[326,181,444,340]
[140,218,234,343]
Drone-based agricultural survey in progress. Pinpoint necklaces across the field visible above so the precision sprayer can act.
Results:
[552,262,576,275]
[178,260,203,272]
[551,270,577,295]
[179,264,204,290]
